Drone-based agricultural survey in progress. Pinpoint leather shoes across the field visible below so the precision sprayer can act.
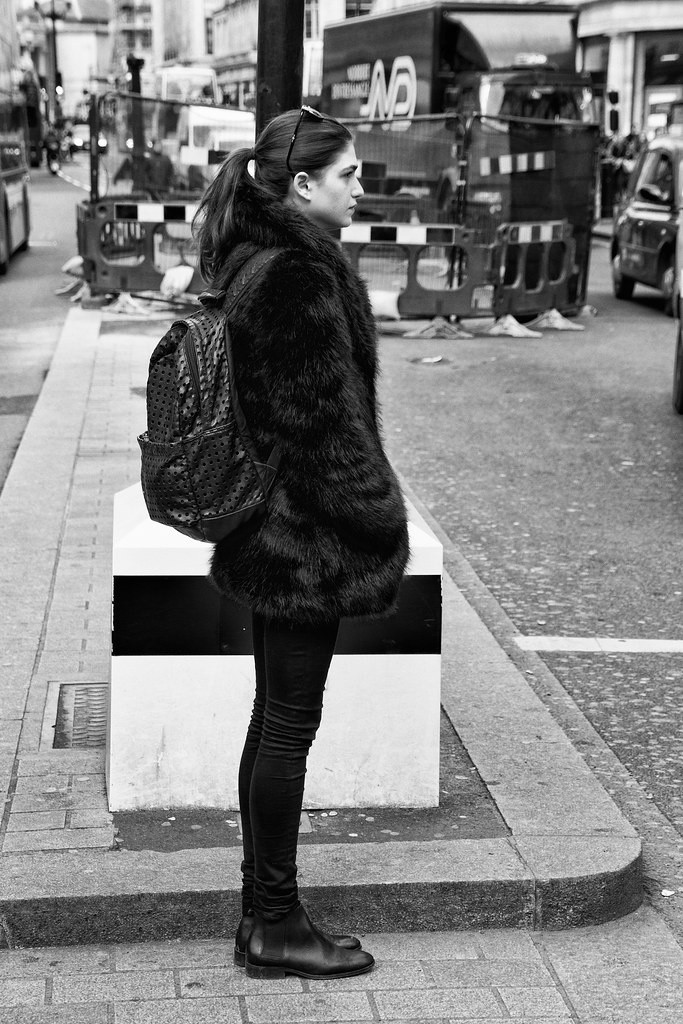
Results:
[245,912,375,981]
[234,910,362,968]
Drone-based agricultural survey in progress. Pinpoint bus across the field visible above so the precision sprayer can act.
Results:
[150,66,226,155]
[0,2,32,274]
[116,71,157,153]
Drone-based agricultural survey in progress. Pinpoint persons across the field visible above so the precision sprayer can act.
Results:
[44,129,59,165]
[193,104,409,980]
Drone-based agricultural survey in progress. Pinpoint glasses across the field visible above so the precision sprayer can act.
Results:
[286,104,323,179]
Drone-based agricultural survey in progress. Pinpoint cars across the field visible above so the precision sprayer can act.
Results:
[154,103,255,185]
[68,124,110,153]
[609,135,683,324]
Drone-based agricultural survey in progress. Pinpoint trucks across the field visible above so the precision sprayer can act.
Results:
[322,0,619,227]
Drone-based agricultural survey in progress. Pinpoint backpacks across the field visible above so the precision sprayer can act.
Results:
[137,247,280,543]
